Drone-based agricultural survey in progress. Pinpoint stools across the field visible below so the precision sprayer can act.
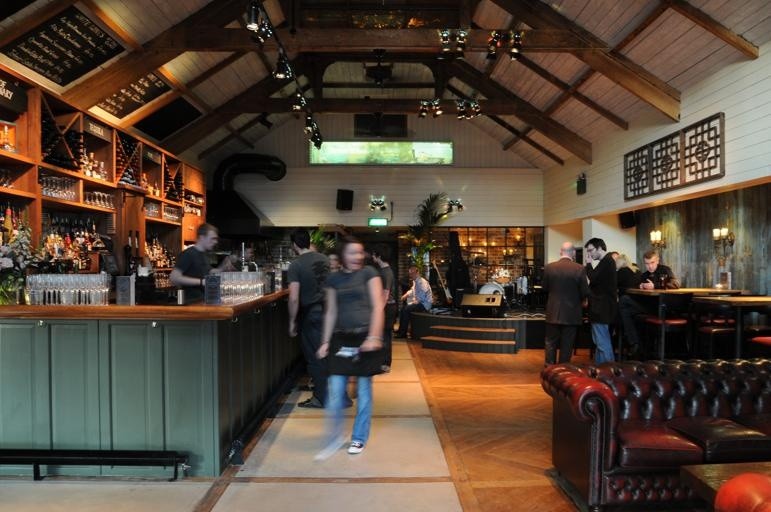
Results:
[751,336,771,358]
[647,318,689,357]
[693,327,735,362]
[744,323,769,335]
[702,319,736,340]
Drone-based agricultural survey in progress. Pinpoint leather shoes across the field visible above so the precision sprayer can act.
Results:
[393,335,406,338]
[628,343,641,356]
[392,330,400,333]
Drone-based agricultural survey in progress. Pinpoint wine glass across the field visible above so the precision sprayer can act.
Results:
[42,177,76,201]
[84,191,115,209]
[220,272,265,305]
[154,273,172,289]
[26,273,111,306]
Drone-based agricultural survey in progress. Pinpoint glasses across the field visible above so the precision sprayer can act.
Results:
[586,247,596,253]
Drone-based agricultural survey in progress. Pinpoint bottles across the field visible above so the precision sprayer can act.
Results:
[145,202,182,223]
[146,234,176,268]
[42,109,187,202]
[46,217,95,270]
[0,201,26,246]
[274,265,282,289]
[0,125,16,151]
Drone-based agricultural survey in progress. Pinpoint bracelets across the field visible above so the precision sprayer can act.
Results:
[200,278,202,286]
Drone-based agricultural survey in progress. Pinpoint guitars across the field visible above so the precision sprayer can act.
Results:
[431,259,455,307]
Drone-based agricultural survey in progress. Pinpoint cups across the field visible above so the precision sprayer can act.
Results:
[659,274,667,289]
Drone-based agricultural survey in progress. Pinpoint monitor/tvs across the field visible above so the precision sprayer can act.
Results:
[354,114,408,137]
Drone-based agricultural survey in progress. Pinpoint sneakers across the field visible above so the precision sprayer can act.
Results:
[299,395,322,408]
[348,440,366,454]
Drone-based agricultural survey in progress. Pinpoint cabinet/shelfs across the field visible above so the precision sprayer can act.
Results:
[408,227,544,292]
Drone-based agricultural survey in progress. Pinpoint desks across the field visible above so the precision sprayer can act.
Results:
[682,461,771,512]
[695,296,771,359]
[626,288,750,360]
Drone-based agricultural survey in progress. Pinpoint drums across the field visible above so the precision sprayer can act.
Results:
[493,270,512,282]
[480,281,505,294]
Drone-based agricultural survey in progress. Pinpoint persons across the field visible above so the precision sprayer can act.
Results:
[540,241,592,369]
[584,237,618,364]
[609,250,679,357]
[393,267,432,339]
[234,247,256,272]
[168,222,218,307]
[311,236,385,461]
[285,229,397,408]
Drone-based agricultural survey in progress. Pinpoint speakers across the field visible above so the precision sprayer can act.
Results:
[618,211,635,229]
[461,294,505,317]
[336,188,354,210]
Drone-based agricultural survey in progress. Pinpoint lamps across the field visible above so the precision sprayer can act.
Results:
[431,27,521,63]
[712,227,735,265]
[648,230,666,250]
[414,91,482,122]
[247,8,323,150]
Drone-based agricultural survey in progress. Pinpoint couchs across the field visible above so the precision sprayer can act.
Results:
[713,475,771,512]
[540,357,771,512]
